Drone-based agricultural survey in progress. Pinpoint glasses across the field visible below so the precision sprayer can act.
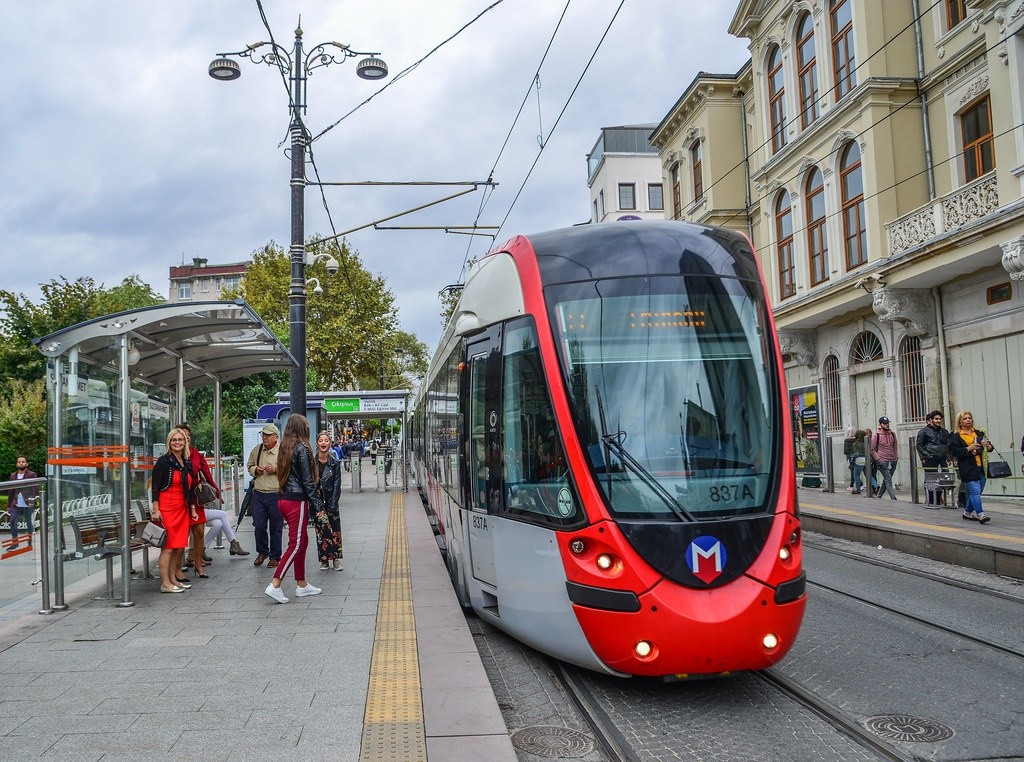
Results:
[260,433,274,438]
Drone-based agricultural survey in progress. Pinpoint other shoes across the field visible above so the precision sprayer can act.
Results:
[846,487,853,490]
[852,489,860,493]
[255,553,268,565]
[977,515,990,524]
[860,486,864,491]
[963,514,979,521]
[161,583,185,593]
[171,580,191,589]
[267,558,278,567]
[875,486,880,495]
[7,545,19,552]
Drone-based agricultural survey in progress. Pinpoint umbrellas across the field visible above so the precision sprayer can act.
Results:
[234,464,257,532]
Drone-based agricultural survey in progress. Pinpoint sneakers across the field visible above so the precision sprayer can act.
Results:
[265,583,289,604]
[320,560,329,570]
[295,582,322,597]
[333,559,343,571]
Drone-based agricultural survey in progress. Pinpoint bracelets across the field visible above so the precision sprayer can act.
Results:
[191,505,195,508]
[152,511,157,514]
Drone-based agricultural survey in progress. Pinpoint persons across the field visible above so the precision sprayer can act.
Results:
[309,432,343,570]
[176,424,250,578]
[264,413,325,604]
[916,410,967,508]
[843,427,880,495]
[7,456,38,551]
[870,416,898,500]
[247,425,284,567]
[151,428,199,593]
[332,439,380,472]
[949,411,994,524]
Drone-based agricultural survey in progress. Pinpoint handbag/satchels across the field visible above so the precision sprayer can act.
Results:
[987,445,1012,478]
[141,518,167,548]
[194,470,217,506]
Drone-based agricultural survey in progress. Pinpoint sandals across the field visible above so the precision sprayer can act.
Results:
[185,559,207,567]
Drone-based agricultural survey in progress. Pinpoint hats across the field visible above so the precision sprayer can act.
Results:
[259,425,280,437]
[878,416,891,424]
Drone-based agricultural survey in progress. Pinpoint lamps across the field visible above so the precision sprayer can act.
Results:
[127,343,141,366]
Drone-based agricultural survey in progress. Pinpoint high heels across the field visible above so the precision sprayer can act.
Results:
[175,571,190,581]
[230,542,250,555]
[193,564,209,578]
[202,549,212,561]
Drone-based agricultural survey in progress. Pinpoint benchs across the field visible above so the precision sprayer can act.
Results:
[69,499,187,601]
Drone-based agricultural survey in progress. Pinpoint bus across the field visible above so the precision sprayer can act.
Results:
[401,218,805,679]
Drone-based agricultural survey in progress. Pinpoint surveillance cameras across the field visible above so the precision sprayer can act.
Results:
[314,286,323,295]
[326,259,339,274]
[854,277,868,289]
[870,273,884,280]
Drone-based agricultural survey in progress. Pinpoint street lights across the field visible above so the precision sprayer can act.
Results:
[210,13,388,415]
